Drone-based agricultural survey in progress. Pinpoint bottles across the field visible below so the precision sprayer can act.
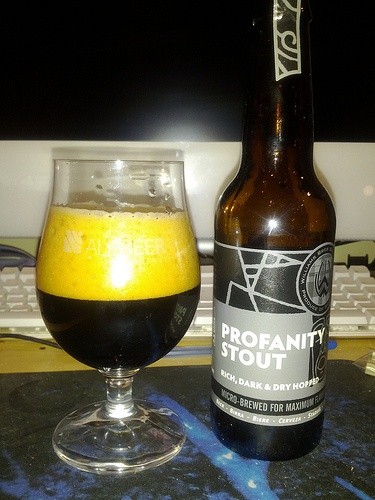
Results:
[209,0,337,461]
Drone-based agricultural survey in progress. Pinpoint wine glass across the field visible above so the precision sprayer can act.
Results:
[35,159,201,475]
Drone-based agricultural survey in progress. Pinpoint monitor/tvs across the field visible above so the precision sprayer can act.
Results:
[0,0,375,253]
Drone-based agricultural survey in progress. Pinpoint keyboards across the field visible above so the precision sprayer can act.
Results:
[0,265,375,341]
